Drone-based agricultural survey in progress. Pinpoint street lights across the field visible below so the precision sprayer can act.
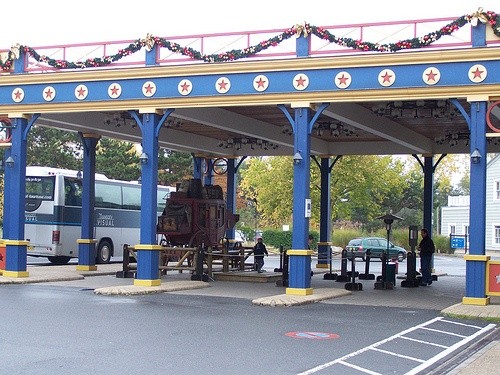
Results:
[375,208,404,289]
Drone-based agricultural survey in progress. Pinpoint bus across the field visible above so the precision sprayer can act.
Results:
[23,166,186,266]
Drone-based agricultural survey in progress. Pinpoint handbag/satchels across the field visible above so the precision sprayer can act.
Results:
[165,204,188,216]
[178,179,189,192]
[202,185,224,199]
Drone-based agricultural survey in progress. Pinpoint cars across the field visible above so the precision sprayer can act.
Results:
[345,237,408,263]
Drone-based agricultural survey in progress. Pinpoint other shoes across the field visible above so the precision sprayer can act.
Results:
[428,281,432,285]
[258,270,262,273]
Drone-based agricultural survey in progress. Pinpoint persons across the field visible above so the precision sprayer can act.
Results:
[416,229,436,286]
[308,234,314,249]
[253,238,268,273]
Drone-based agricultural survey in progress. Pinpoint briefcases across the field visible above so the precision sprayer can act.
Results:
[189,178,202,198]
[158,215,180,231]
